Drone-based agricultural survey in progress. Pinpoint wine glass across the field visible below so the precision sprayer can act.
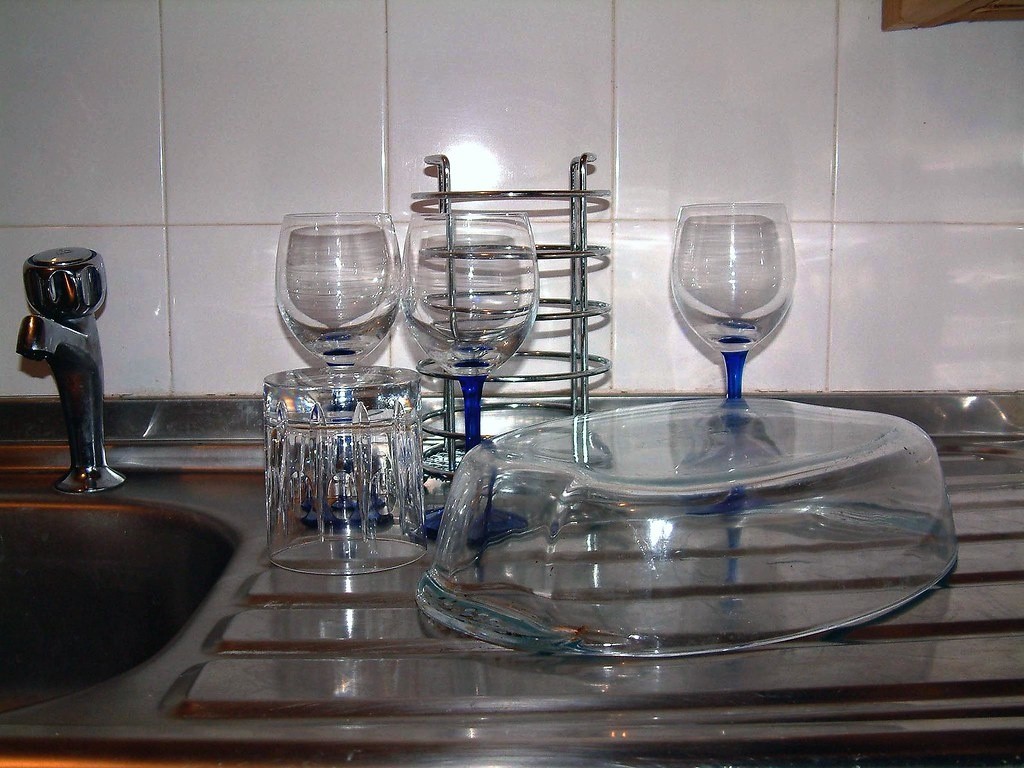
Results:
[667,201,800,421]
[399,209,530,544]
[276,212,404,528]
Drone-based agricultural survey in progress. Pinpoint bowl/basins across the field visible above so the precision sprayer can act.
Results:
[415,396,961,662]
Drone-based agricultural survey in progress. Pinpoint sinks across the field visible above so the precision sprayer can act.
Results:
[1,469,245,720]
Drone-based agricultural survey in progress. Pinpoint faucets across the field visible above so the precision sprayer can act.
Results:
[12,245,128,494]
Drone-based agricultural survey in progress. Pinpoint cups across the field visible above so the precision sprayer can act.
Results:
[261,366,430,577]
